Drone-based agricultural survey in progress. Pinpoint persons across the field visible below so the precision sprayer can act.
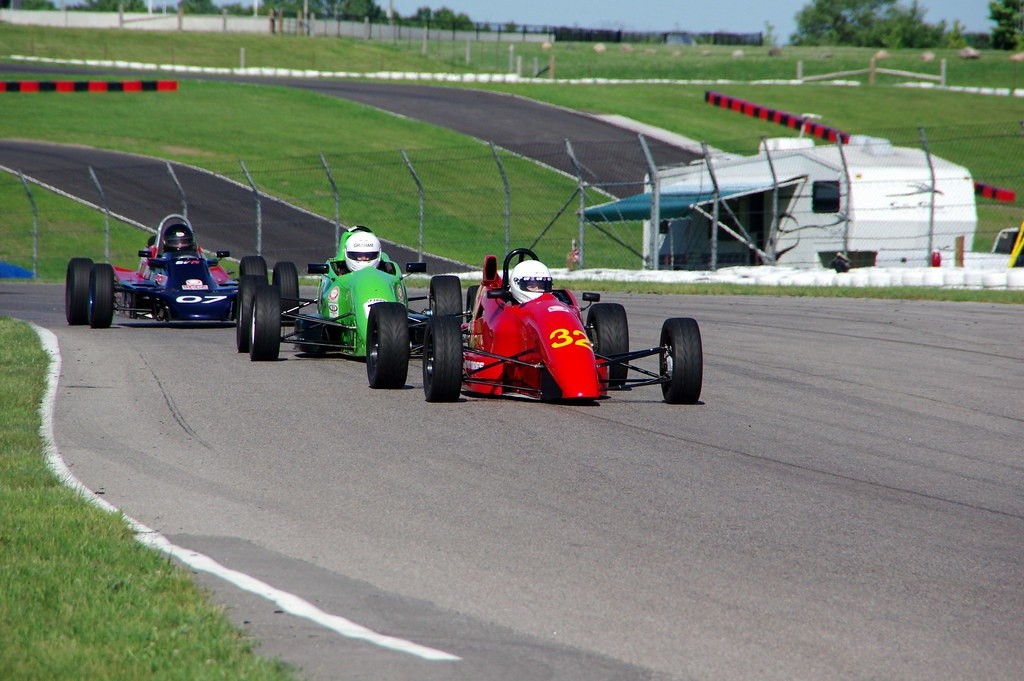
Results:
[343,231,382,272]
[505,259,554,305]
[163,224,193,254]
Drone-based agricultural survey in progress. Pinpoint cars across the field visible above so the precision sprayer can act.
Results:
[959,46,980,57]
[993,224,1023,268]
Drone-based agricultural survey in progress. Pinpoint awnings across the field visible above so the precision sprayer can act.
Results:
[576,172,809,262]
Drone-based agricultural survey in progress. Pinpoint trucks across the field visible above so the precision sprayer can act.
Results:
[576,118,978,269]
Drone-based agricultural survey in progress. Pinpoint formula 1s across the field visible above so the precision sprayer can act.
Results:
[233,225,480,364]
[64,215,305,329]
[366,245,707,405]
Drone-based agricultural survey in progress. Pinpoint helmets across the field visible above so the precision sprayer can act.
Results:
[163,224,194,254]
[507,258,553,304]
[344,230,382,271]
